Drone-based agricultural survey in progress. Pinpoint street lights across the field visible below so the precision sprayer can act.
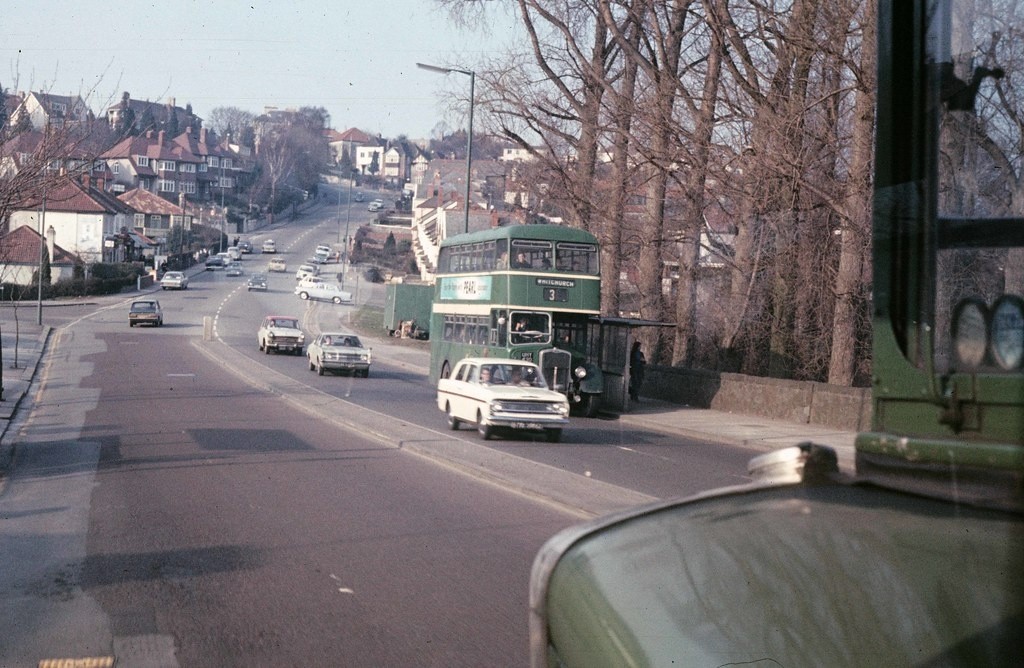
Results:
[416,63,475,234]
[342,166,358,273]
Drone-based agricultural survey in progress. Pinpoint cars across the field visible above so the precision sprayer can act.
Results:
[295,282,352,303]
[355,192,364,202]
[307,333,372,378]
[295,245,332,281]
[257,315,305,355]
[436,356,570,444]
[247,273,268,292]
[129,299,163,327]
[159,271,188,290]
[204,239,286,276]
[368,199,384,214]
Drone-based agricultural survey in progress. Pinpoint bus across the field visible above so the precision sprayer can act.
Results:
[429,224,603,420]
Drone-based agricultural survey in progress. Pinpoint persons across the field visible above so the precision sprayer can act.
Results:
[322,335,334,346]
[513,252,532,269]
[516,316,531,342]
[234,237,237,247]
[480,368,494,386]
[555,257,566,272]
[507,367,531,386]
[269,320,275,328]
[343,338,354,347]
[629,341,647,404]
[541,259,552,272]
[162,259,167,272]
[237,236,240,244]
[496,250,508,268]
